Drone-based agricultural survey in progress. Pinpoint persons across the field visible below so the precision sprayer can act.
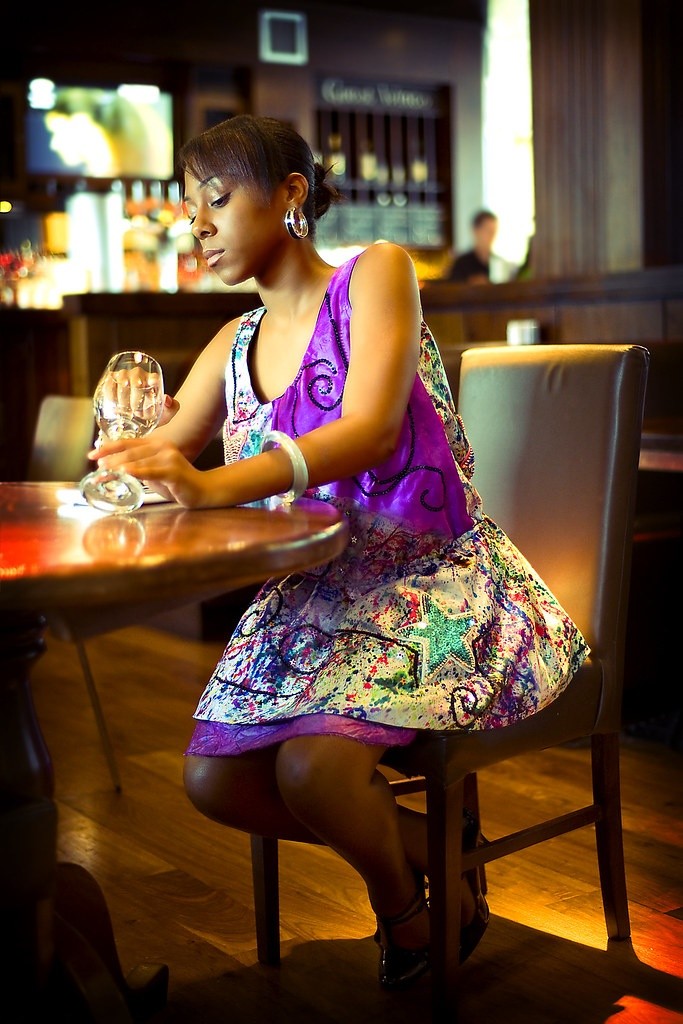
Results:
[445,208,518,284]
[86,115,591,989]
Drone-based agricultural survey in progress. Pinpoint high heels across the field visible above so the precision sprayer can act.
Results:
[437,815,490,965]
[370,860,448,995]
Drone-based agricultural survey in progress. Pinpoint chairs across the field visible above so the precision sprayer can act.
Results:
[31,394,124,796]
[249,344,651,1024]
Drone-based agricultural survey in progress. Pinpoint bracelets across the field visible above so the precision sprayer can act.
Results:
[259,430,308,503]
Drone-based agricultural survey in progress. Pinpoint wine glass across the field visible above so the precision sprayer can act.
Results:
[80,350,163,513]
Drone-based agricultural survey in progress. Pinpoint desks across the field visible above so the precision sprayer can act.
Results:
[637,413,683,473]
[0,480,352,1024]
